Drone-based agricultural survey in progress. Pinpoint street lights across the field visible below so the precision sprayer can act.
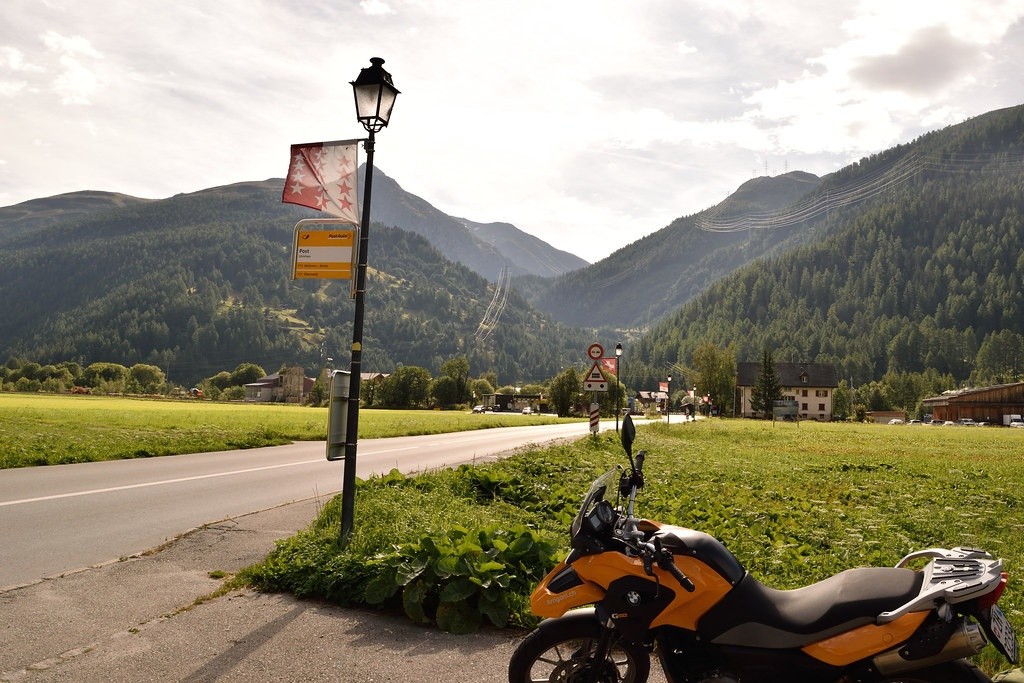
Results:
[707,392,710,417]
[667,373,672,423]
[338,55,402,544]
[693,385,697,421]
[613,341,623,433]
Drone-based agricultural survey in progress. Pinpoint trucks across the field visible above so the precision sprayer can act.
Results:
[1002,414,1022,427]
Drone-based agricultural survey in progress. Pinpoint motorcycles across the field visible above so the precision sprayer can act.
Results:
[508,406,1020,683]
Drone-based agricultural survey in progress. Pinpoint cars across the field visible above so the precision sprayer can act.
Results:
[472,404,485,413]
[493,405,502,412]
[887,417,991,427]
[522,407,531,414]
